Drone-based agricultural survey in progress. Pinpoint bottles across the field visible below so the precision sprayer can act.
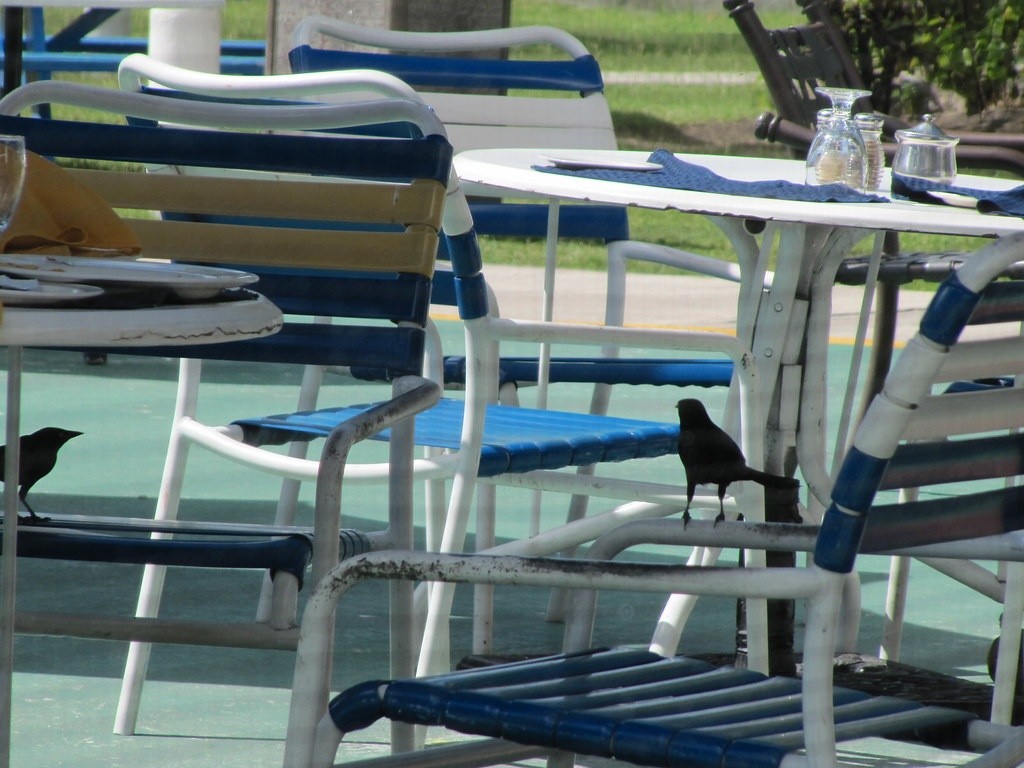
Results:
[854,113,886,191]
[817,108,851,184]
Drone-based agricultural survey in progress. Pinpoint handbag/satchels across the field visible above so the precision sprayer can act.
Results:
[0,141,142,256]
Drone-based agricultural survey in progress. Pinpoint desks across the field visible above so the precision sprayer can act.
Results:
[410,149,1024,768]
[0,275,286,768]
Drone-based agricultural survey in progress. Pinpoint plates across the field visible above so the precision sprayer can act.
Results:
[0,253,259,299]
[538,153,663,173]
[0,279,104,306]
[926,190,978,208]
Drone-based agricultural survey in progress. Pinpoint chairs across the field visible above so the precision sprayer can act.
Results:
[0,0,1024,768]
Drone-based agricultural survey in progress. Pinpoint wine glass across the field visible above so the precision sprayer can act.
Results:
[806,86,873,195]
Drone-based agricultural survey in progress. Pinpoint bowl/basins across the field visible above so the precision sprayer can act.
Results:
[891,113,961,186]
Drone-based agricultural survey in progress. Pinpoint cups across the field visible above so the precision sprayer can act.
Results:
[0,134,27,233]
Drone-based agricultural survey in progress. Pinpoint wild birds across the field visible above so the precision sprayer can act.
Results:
[675,398,800,531]
[0,427,84,521]
